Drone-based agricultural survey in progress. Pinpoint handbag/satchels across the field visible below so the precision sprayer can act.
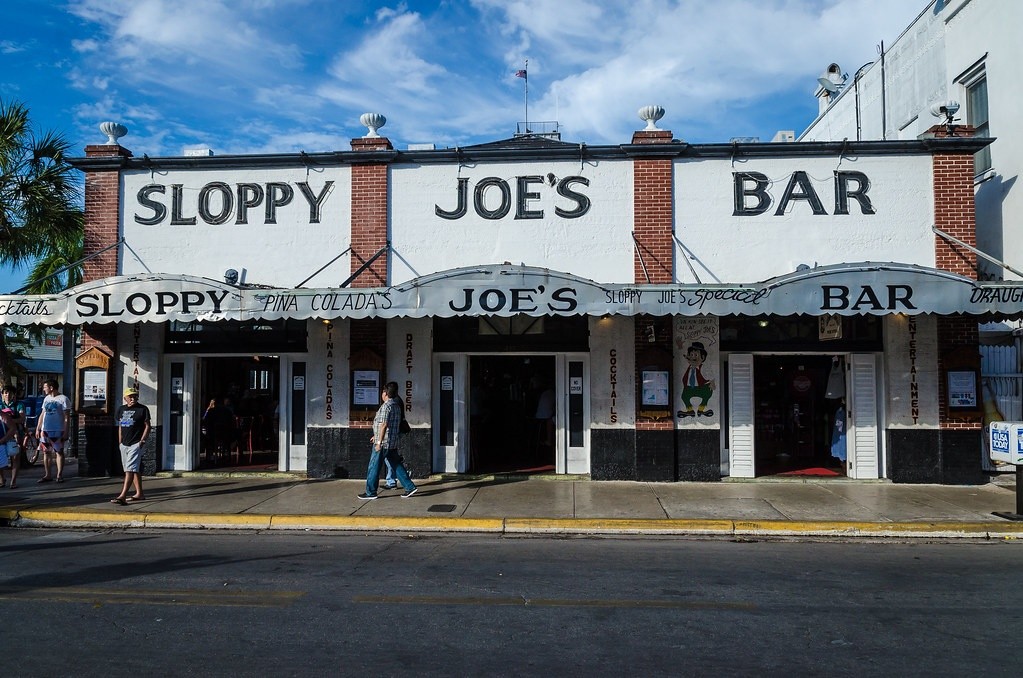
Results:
[6,440,19,456]
[399,420,411,434]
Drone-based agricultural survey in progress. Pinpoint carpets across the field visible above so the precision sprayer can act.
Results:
[755,466,841,476]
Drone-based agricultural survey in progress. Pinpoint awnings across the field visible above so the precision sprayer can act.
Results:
[0,261,1023,326]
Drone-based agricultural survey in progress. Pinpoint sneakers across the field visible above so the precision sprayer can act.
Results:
[357,493,378,499]
[401,486,418,498]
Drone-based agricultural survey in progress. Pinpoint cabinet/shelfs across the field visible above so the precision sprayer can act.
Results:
[754,387,814,460]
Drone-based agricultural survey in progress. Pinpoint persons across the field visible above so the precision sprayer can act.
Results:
[111,388,151,504]
[357,382,418,499]
[0,386,26,489]
[267,400,279,440]
[35,379,71,483]
[535,389,578,447]
[200,398,233,448]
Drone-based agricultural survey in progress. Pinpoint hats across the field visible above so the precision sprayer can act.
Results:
[123,387,139,397]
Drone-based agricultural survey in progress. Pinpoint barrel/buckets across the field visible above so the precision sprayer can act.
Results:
[18,400,36,427]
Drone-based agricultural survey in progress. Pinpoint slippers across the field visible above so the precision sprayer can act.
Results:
[37,476,53,483]
[125,496,145,501]
[56,475,63,483]
[111,498,128,505]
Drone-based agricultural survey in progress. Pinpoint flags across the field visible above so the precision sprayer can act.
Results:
[516,70,526,79]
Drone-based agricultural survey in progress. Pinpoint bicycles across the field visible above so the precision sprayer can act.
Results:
[15,413,43,467]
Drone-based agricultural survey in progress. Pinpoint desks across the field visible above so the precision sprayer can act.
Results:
[203,416,255,465]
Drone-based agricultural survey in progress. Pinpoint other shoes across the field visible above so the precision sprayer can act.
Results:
[380,483,397,490]
[0,479,7,488]
[10,482,16,489]
[407,470,413,481]
